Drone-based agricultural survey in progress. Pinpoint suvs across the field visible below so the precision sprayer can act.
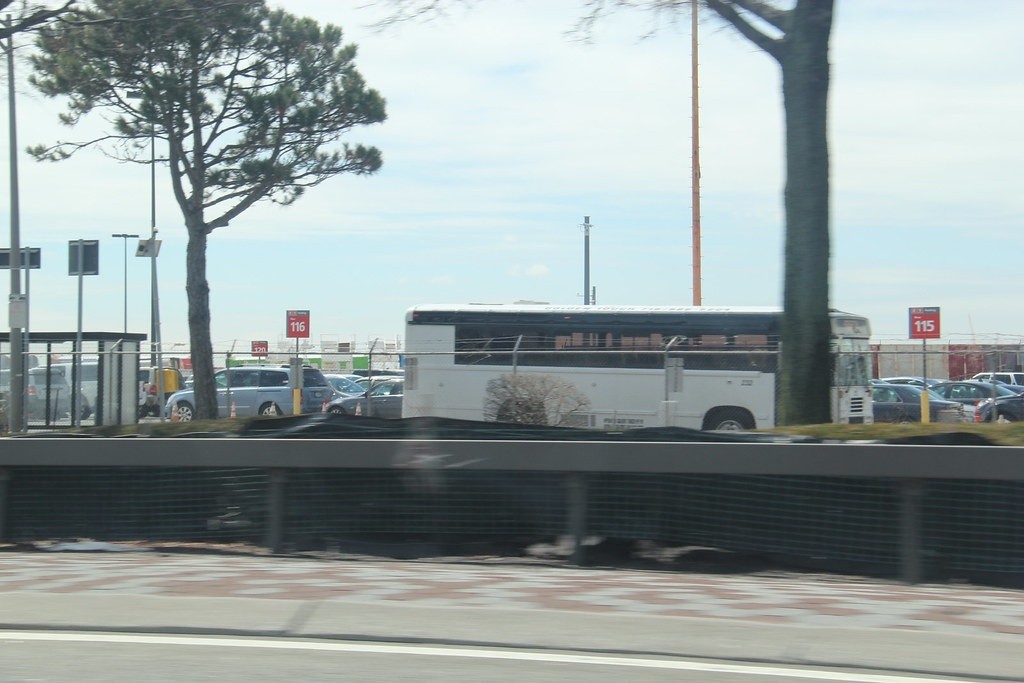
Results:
[165,363,332,423]
[0,363,197,421]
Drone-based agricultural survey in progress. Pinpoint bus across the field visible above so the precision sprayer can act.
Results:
[402,298,875,432]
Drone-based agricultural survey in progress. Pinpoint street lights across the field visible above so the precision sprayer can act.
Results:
[110,234,139,334]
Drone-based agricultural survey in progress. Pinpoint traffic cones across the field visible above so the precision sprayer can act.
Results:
[353,402,363,417]
[229,402,238,417]
[320,401,328,415]
[170,405,180,422]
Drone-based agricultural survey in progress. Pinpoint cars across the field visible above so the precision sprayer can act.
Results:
[869,372,1024,425]
[321,368,404,420]
